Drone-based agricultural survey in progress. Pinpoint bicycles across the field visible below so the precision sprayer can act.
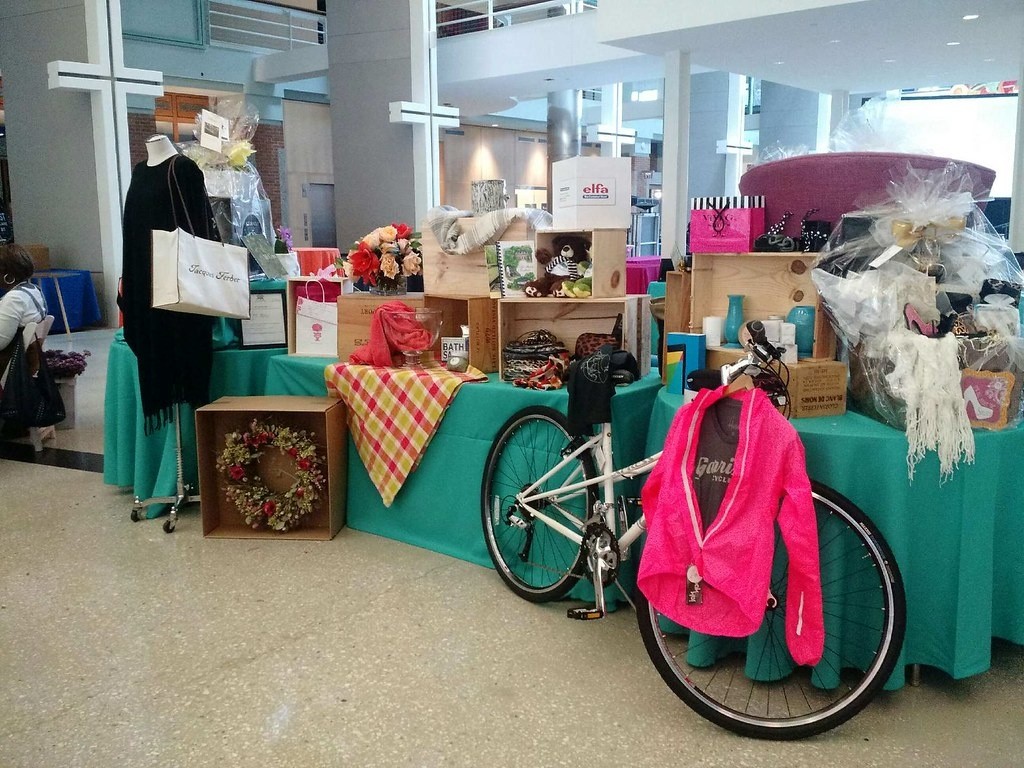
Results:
[481,319,908,741]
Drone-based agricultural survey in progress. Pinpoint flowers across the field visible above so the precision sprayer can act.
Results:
[215,413,329,532]
[333,222,426,291]
[43,347,92,377]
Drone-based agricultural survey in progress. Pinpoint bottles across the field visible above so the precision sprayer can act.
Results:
[723,294,744,348]
[785,306,815,358]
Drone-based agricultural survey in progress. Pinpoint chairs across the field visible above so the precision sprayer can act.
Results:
[0,314,57,452]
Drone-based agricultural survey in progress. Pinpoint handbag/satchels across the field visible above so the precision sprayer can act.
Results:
[296,280,339,356]
[150,154,251,321]
[0,327,66,427]
[689,195,765,253]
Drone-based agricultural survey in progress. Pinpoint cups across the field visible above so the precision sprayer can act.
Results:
[470,178,510,217]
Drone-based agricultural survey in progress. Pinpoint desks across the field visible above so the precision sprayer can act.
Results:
[26,269,102,333]
[103,324,288,503]
[626,256,675,294]
[640,383,1024,692]
[292,246,340,276]
[265,352,663,612]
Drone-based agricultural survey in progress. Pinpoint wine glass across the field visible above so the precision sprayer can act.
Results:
[381,306,442,370]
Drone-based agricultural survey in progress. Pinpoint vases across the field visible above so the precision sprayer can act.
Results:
[367,265,408,295]
[473,179,506,217]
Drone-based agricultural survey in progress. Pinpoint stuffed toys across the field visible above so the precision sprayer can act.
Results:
[524,235,593,298]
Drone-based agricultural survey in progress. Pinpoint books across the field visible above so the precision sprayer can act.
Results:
[482,240,534,300]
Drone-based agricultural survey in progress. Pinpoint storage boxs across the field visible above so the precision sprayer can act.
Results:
[286,276,354,357]
[553,155,631,229]
[21,242,51,270]
[194,394,346,540]
[423,294,499,374]
[689,251,838,362]
[534,230,627,299]
[705,349,847,420]
[497,296,638,380]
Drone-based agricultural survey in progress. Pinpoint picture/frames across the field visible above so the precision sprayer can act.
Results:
[238,290,289,349]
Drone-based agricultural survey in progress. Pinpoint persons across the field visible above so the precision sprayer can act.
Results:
[0,242,49,441]
[114,134,226,535]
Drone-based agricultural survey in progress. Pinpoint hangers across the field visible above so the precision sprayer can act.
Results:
[723,358,760,398]
